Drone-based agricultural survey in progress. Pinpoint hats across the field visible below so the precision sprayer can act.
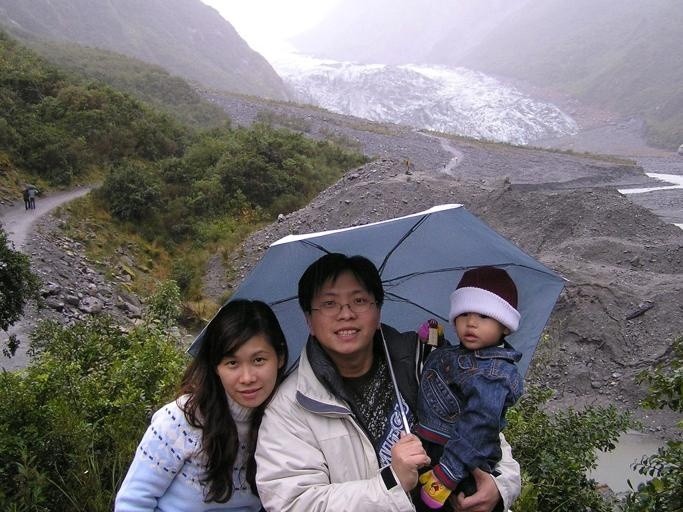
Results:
[449,266,522,333]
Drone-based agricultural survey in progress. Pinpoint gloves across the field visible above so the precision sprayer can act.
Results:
[418,319,445,347]
[419,463,458,509]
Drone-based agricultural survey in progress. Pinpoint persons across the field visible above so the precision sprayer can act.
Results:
[22,187,31,210]
[254,252,522,511]
[408,263,527,511]
[27,186,36,209]
[112,296,289,511]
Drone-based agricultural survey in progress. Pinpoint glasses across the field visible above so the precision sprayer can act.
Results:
[312,297,378,317]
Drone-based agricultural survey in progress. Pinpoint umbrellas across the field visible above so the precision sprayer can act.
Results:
[184,200,575,471]
